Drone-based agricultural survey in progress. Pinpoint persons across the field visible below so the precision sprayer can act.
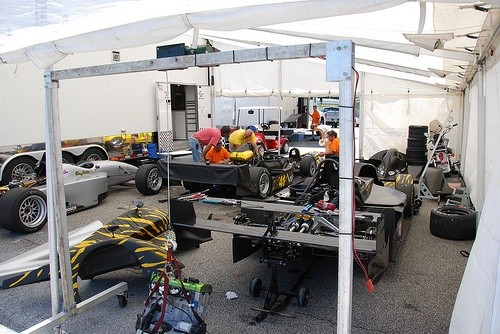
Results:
[206,142,231,164]
[189,124,230,162]
[226,128,259,157]
[312,124,333,152]
[318,130,339,157]
[307,106,321,141]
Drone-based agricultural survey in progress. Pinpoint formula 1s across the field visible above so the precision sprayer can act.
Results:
[0,150,423,261]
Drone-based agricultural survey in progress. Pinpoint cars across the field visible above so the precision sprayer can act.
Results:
[319,106,359,126]
